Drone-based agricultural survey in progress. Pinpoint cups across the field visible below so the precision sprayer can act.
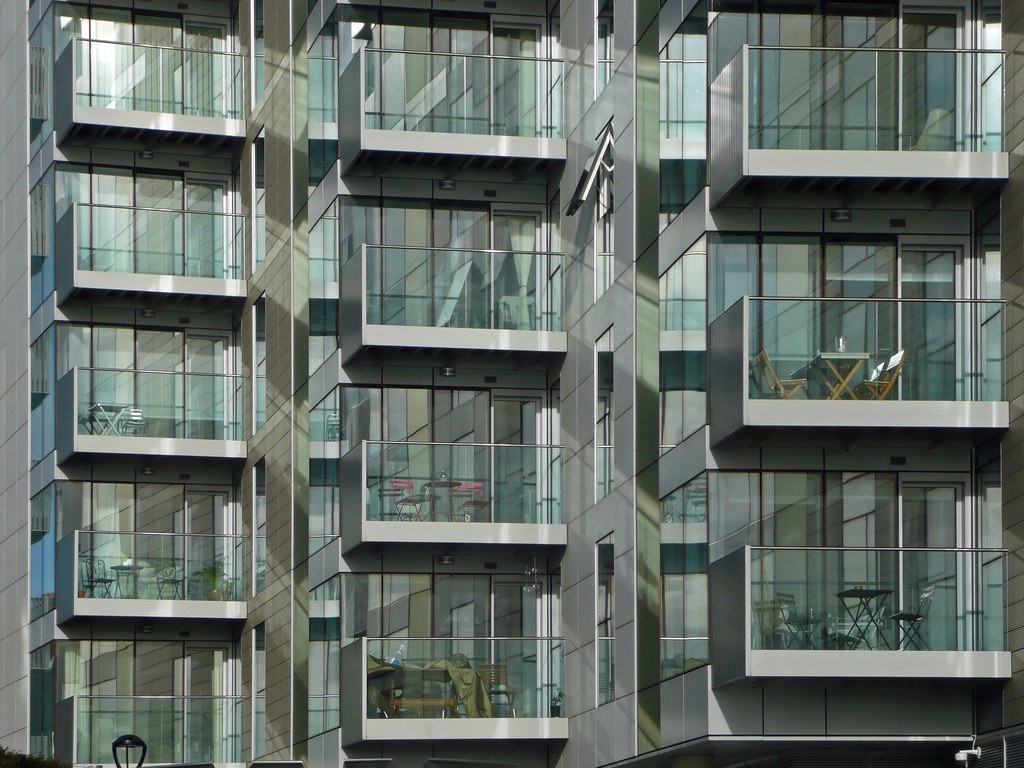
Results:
[464,515,470,522]
[853,585,863,589]
[667,515,672,523]
[779,605,789,620]
[127,559,134,565]
[122,562,128,566]
[834,335,848,353]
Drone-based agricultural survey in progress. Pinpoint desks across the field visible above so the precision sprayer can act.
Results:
[808,351,869,400]
[790,364,826,400]
[389,668,478,718]
[831,587,894,650]
[110,564,143,599]
[458,481,490,515]
[79,410,109,436]
[89,402,128,436]
[425,480,460,522]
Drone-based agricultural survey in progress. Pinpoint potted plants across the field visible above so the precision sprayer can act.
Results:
[193,557,230,601]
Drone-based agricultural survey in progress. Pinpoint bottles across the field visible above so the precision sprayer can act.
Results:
[439,470,447,481]
[385,643,407,666]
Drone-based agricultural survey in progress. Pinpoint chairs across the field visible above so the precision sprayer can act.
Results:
[903,108,954,151]
[85,556,115,597]
[121,409,149,436]
[854,350,906,399]
[391,478,424,522]
[478,663,516,718]
[869,362,885,380]
[148,560,183,600]
[757,348,807,400]
[889,584,936,651]
[777,592,820,650]
